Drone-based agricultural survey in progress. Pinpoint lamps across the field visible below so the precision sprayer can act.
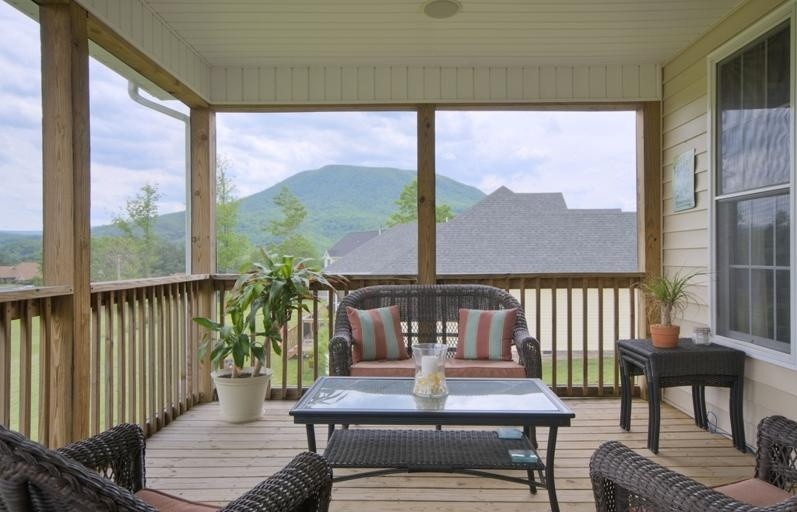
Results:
[421,1,462,20]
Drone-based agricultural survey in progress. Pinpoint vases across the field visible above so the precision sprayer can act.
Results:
[412,343,449,398]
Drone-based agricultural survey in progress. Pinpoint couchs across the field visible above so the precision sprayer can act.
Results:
[328,285,542,449]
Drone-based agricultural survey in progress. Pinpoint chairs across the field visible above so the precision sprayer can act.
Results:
[0,422,333,512]
[589,415,797,512]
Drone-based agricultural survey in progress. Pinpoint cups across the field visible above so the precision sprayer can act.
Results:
[693,326,713,346]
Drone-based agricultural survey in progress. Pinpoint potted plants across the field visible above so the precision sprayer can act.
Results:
[630,262,715,348]
[191,247,350,425]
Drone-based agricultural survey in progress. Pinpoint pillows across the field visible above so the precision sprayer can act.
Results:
[454,307,519,361]
[346,304,410,364]
